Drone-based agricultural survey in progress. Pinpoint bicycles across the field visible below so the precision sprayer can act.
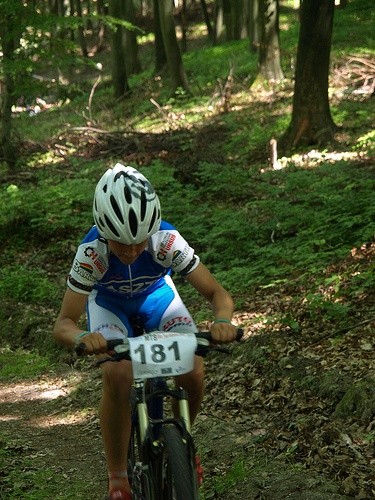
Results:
[74,324,246,500]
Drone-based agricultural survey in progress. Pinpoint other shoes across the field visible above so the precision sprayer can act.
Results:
[109,475,131,499]
[195,454,202,486]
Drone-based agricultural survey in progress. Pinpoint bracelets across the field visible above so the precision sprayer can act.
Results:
[75,332,90,346]
[213,319,231,325]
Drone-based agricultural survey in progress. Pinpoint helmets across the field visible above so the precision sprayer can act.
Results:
[93,163,161,245]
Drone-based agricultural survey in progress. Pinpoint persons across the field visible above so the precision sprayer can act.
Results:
[53,164,235,500]
